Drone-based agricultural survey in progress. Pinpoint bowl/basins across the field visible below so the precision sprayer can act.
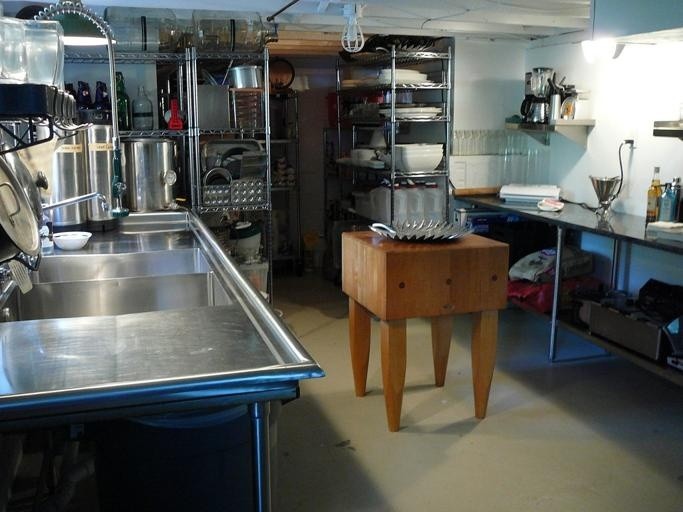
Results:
[52,230,92,251]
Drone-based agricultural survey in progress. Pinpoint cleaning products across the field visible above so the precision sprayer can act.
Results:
[654,182,677,222]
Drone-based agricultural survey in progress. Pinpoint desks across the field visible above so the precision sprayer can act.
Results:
[341,227,511,435]
[451,189,682,392]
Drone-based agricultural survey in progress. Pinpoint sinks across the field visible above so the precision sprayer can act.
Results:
[118,221,192,234]
[27,246,213,282]
[119,212,189,222]
[0,280,234,324]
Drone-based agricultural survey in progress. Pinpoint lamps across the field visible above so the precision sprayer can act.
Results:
[338,4,367,55]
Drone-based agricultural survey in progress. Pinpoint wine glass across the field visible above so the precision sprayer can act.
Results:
[589,175,621,217]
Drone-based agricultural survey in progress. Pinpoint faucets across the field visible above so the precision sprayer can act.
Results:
[42,191,109,212]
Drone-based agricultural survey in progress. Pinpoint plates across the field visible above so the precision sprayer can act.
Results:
[340,65,448,176]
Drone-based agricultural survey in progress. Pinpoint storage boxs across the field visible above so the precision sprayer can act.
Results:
[586,299,682,363]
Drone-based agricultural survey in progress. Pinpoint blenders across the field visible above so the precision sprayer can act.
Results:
[520,67,554,124]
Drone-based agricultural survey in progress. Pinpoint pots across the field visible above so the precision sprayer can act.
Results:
[229,62,264,91]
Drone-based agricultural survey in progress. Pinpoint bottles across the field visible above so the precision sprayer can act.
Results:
[132,84,153,130]
[645,166,664,225]
[114,70,131,131]
[64,71,111,126]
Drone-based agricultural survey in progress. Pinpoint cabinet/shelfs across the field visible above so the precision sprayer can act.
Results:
[324,49,452,269]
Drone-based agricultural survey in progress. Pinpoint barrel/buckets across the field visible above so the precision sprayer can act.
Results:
[370,180,447,225]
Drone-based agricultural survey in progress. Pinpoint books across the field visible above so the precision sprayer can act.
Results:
[497,181,562,205]
[645,220,683,233]
[656,232,683,241]
